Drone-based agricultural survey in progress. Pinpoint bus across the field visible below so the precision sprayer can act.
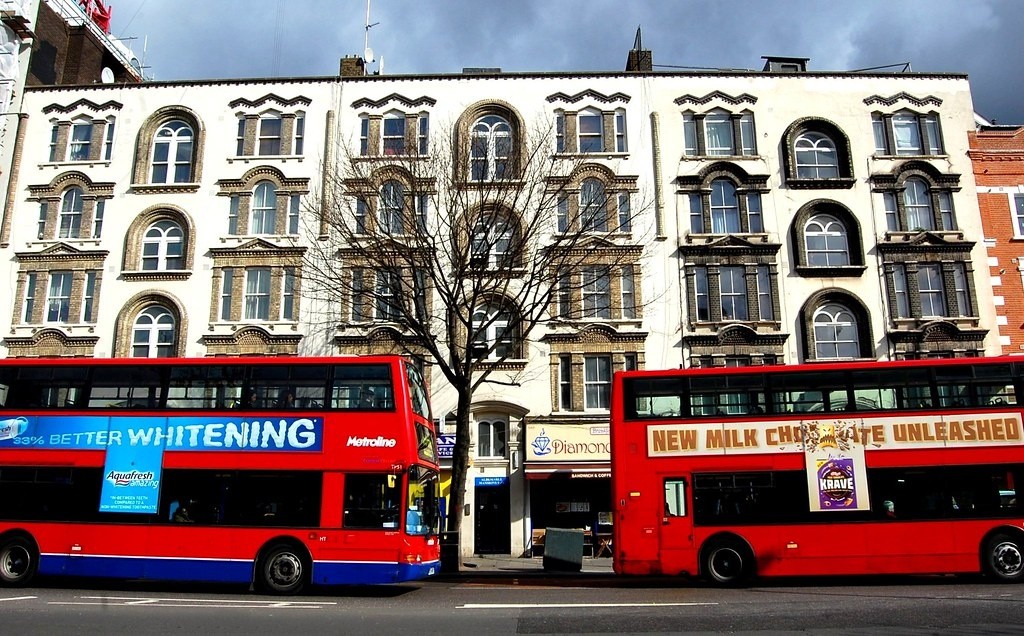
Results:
[0,358,443,595]
[611,356,1024,588]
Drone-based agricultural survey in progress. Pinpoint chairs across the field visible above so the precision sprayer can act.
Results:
[920,397,1009,408]
[311,400,324,409]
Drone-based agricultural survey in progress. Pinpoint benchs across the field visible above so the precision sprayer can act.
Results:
[532,528,595,560]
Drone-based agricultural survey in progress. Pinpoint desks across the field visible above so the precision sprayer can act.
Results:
[594,533,613,557]
